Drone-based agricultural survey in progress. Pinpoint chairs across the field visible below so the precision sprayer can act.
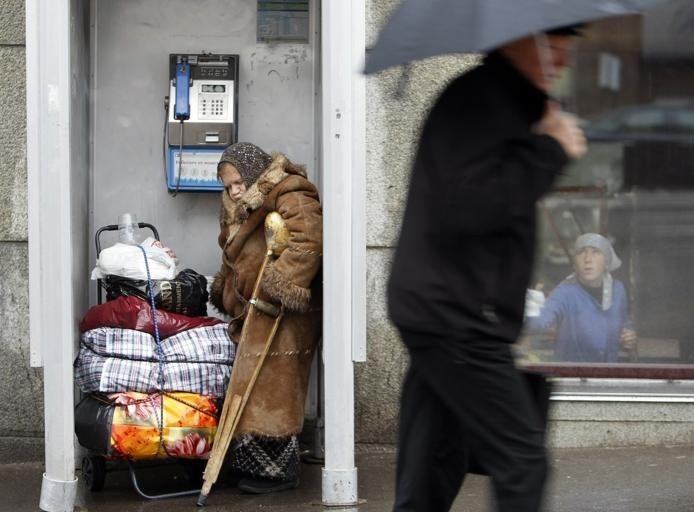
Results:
[239,477,298,492]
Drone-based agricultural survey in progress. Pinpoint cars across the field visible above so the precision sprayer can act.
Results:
[117,212,141,247]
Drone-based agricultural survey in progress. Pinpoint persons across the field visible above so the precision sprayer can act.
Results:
[210,141,322,495]
[383,21,589,511]
[522,234,638,359]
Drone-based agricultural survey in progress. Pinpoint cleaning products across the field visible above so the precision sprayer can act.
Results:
[81,219,225,499]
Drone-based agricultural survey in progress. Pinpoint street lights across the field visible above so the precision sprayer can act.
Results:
[74,236,236,461]
[404,358,551,483]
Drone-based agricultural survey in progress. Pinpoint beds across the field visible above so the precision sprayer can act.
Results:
[359,1,666,121]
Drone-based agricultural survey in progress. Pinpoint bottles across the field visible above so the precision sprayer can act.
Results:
[543,26,587,39]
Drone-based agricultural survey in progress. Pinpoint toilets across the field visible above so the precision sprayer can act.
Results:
[169,54,240,149]
